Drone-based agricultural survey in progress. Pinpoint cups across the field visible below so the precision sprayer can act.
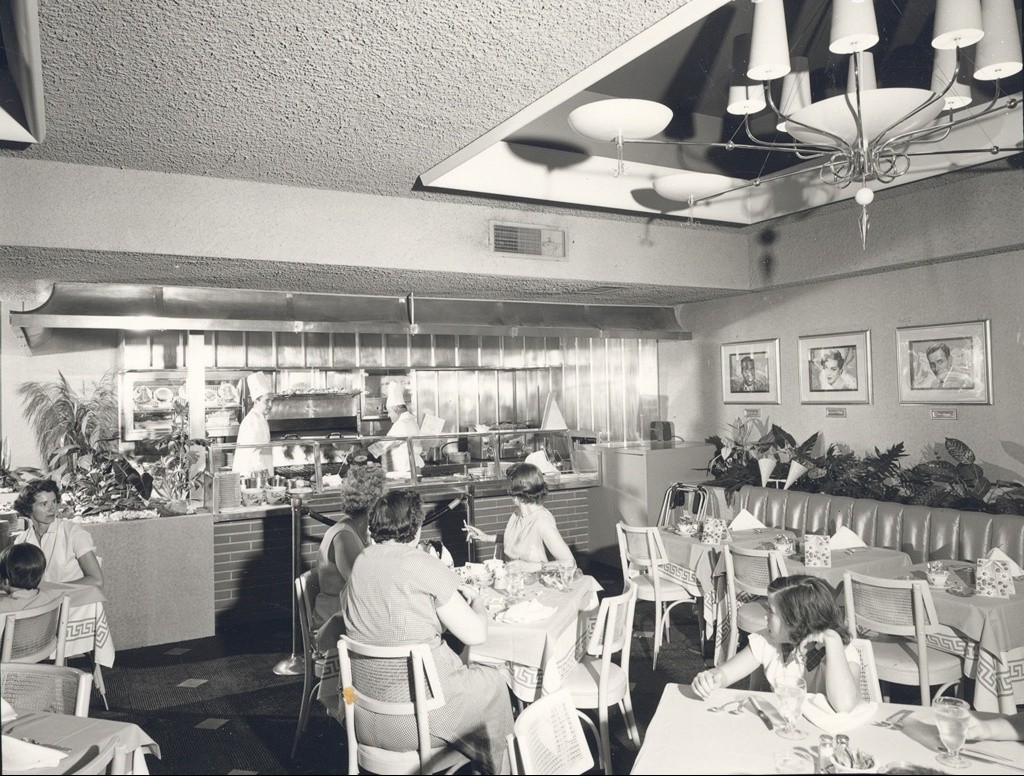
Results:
[482,558,503,571]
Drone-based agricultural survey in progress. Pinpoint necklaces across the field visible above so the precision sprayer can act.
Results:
[351,517,368,544]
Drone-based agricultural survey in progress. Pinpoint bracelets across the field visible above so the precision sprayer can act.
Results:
[540,560,547,575]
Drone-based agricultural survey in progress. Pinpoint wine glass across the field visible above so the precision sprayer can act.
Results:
[931,695,972,769]
[502,565,521,602]
[774,675,808,740]
[557,558,577,593]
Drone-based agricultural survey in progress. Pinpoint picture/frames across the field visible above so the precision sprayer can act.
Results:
[720,338,781,405]
[894,319,993,406]
[796,329,873,405]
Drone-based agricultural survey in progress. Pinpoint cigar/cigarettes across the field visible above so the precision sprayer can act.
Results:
[463,520,470,533]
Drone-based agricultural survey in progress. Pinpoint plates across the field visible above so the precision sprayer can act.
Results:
[134,383,235,402]
[929,586,952,592]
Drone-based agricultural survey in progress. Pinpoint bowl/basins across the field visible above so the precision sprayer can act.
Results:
[242,488,264,506]
[925,569,949,586]
[287,487,313,500]
[264,486,287,504]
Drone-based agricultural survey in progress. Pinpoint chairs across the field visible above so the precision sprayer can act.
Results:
[0,485,1024,775]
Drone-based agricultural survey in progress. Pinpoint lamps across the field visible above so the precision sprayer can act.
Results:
[565,0,1024,249]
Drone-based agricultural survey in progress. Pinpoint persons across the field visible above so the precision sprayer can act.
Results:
[0,542,58,661]
[314,461,388,633]
[818,352,857,390]
[0,477,104,590]
[340,486,515,775]
[462,462,577,574]
[920,344,974,389]
[741,356,767,393]
[233,371,275,485]
[691,574,861,713]
[348,381,425,479]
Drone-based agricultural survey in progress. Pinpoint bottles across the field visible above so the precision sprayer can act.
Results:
[789,539,800,554]
[818,734,849,773]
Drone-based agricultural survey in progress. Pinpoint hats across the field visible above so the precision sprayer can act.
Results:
[247,371,273,401]
[386,381,405,407]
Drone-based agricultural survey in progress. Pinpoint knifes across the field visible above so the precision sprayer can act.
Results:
[747,696,773,731]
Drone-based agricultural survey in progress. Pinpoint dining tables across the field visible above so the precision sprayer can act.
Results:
[628,681,1024,775]
[1,712,162,775]
[699,545,913,663]
[445,563,605,713]
[37,580,114,710]
[625,517,796,662]
[870,557,1024,715]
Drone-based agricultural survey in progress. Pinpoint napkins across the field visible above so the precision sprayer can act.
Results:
[827,526,868,549]
[730,509,767,529]
[988,547,1024,578]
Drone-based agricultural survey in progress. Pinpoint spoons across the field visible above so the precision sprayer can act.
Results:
[708,700,747,714]
[876,709,914,729]
